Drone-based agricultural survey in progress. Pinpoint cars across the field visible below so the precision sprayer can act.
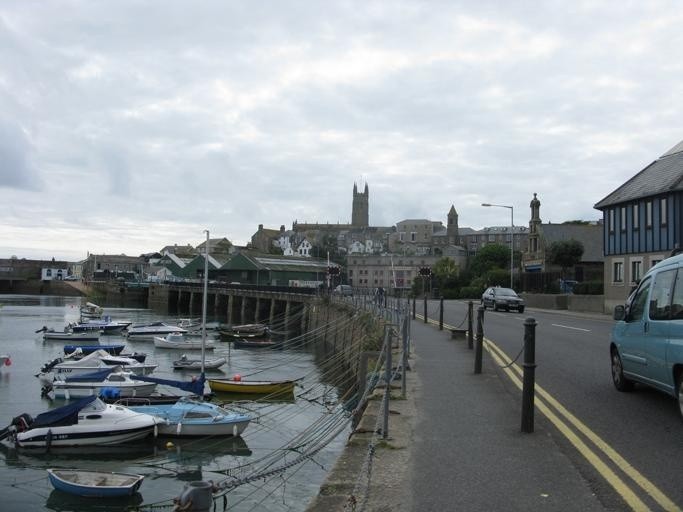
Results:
[115,277,126,282]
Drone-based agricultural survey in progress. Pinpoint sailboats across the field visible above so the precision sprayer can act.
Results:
[33,228,228,403]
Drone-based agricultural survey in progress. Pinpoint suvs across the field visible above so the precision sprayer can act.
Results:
[480,288,526,315]
[559,281,578,294]
[332,285,354,297]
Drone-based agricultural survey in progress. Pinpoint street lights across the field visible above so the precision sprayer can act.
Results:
[480,202,514,291]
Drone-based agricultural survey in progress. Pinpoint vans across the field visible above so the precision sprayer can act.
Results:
[608,248,683,422]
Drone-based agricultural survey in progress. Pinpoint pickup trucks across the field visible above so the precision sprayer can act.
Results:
[64,275,81,281]
